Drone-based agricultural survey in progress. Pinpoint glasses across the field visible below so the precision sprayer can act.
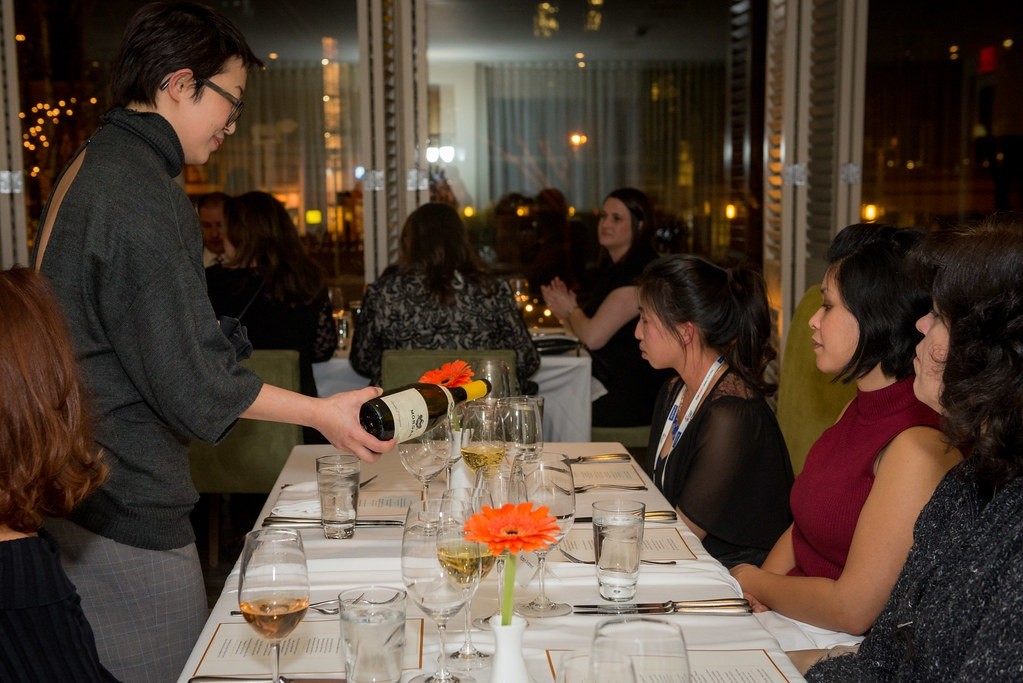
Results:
[159,76,245,128]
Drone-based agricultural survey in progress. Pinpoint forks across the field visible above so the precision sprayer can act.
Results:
[559,546,677,565]
[230,594,364,615]
[548,480,648,496]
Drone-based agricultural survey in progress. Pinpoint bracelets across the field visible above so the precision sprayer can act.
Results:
[566,306,580,322]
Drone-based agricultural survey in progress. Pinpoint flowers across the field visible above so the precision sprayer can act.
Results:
[418,360,474,430]
[464,501,558,623]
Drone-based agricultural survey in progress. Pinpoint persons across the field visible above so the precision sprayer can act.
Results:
[786,221,1022,683]
[495,190,585,294]
[35,0,398,683]
[0,266,122,683]
[635,255,796,567]
[204,190,338,398]
[729,222,973,650]
[350,203,540,393]
[198,190,230,266]
[541,187,669,428]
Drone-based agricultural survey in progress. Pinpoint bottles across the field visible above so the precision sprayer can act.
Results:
[360,378,492,447]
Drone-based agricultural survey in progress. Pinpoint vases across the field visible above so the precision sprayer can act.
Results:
[488,614,530,682]
[451,428,477,499]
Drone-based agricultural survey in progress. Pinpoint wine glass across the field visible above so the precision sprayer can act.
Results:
[238,280,577,683]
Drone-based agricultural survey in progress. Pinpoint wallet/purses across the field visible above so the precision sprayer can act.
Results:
[533,338,582,358]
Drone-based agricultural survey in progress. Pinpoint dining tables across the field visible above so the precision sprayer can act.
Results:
[312,312,592,443]
[176,442,812,682]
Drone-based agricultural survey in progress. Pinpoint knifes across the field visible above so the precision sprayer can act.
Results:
[568,454,631,463]
[263,516,402,524]
[574,510,678,523]
[261,522,402,527]
[573,599,753,615]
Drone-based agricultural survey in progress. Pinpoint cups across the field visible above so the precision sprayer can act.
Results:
[339,587,407,683]
[314,455,360,540]
[591,500,646,602]
[588,616,690,683]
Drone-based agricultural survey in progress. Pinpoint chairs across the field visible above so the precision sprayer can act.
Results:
[188,285,858,596]
